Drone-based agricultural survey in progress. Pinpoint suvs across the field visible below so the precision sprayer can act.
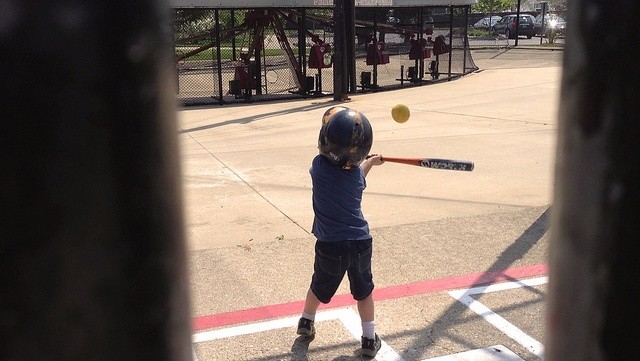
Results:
[493,15,535,39]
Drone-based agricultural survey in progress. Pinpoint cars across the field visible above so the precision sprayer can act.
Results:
[474,17,500,32]
[535,13,567,39]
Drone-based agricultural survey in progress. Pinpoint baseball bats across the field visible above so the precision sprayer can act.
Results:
[367,155,473,172]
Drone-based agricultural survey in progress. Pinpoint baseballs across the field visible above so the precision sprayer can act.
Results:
[391,104,410,123]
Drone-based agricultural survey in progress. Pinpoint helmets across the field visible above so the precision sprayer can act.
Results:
[319,106,373,169]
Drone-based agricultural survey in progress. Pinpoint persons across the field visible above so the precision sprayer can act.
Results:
[296,106,385,358]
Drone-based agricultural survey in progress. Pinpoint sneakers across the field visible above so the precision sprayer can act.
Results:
[296,317,316,336]
[362,333,381,359]
